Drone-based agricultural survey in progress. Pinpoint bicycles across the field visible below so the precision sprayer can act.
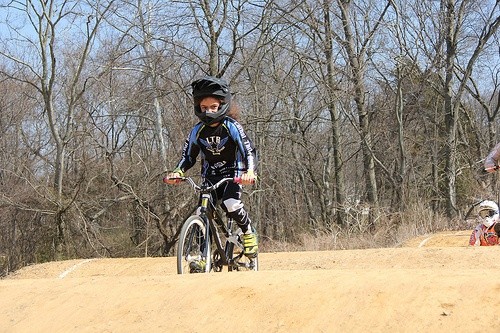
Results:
[167,175,260,276]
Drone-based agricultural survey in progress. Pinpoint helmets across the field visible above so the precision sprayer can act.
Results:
[477,200,499,228]
[191,76,232,124]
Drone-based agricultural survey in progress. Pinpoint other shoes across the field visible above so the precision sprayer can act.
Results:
[242,227,259,257]
[189,259,206,273]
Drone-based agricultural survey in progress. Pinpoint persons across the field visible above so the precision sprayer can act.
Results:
[469,200,500,246]
[163,76,258,272]
